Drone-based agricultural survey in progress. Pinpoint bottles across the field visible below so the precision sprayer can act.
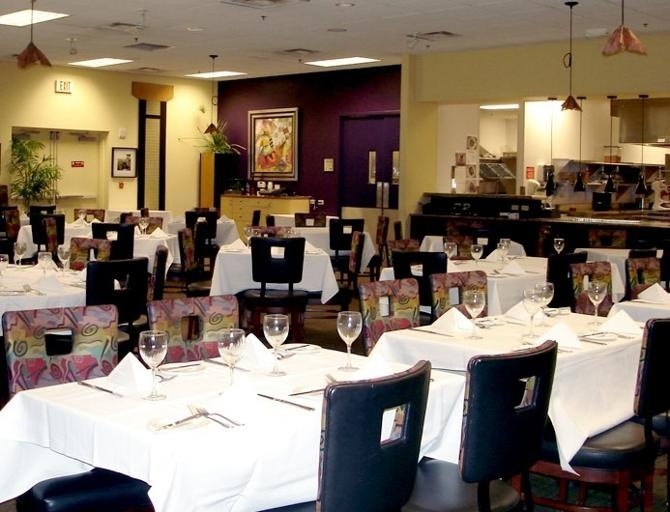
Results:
[244,180,251,195]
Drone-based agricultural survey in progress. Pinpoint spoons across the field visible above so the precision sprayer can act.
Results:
[152,371,177,384]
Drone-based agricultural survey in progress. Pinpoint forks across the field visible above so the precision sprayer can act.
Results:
[543,311,570,318]
[22,285,44,295]
[188,403,241,431]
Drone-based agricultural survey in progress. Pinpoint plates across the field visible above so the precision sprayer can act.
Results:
[281,344,323,355]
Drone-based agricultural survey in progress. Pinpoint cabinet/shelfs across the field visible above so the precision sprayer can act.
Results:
[200,152,234,209]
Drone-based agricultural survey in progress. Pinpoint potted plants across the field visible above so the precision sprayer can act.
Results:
[181,117,246,156]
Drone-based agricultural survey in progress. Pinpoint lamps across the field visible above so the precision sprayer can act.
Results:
[203,54,221,134]
[18,0,52,68]
[604,94,617,191]
[544,97,559,190]
[561,1,582,111]
[574,96,588,190]
[602,0,648,56]
[635,95,648,195]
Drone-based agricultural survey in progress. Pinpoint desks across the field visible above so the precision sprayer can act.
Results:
[1,341,466,512]
[605,284,670,330]
[366,307,656,483]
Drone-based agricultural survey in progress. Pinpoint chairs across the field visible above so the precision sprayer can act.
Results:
[129,344,221,369]
[9,352,155,512]
[399,340,561,512]
[567,318,670,511]
[0,204,670,341]
[315,358,432,512]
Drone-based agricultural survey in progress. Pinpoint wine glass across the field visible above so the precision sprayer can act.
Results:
[535,282,556,327]
[263,313,290,377]
[0,241,71,283]
[138,330,170,401]
[242,225,301,249]
[218,327,245,391]
[588,282,607,332]
[77,209,151,237]
[464,289,486,340]
[521,290,544,340]
[337,311,363,372]
[444,237,566,270]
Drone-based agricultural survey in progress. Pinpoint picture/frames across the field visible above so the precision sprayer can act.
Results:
[111,147,137,178]
[248,107,298,183]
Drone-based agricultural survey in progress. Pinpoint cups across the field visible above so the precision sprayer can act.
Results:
[267,181,274,191]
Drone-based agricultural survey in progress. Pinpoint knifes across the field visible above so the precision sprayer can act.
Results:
[577,332,618,346]
[257,392,313,410]
[159,412,210,430]
[480,315,528,328]
[205,357,252,373]
[160,362,201,371]
[285,345,310,351]
[408,327,454,338]
[77,379,125,400]
[288,390,326,398]
